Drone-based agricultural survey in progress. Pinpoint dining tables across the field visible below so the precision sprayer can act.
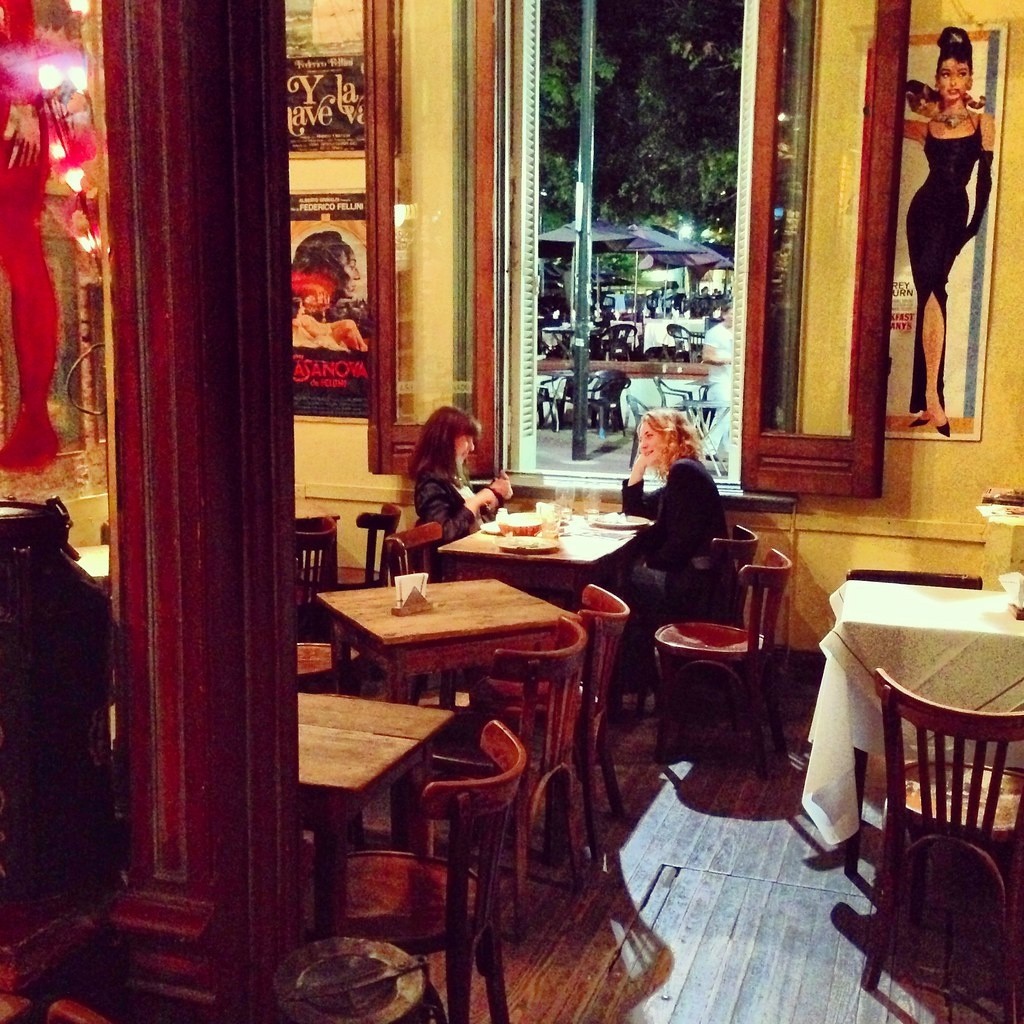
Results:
[295,694,456,942]
[541,325,602,364]
[438,509,659,607]
[316,578,585,843]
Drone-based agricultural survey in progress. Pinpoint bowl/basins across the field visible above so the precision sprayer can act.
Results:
[499,512,546,537]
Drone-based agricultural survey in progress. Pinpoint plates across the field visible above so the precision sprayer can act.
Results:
[481,520,505,534]
[589,515,650,530]
[492,535,562,554]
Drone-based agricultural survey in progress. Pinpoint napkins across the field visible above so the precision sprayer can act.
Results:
[393,572,429,604]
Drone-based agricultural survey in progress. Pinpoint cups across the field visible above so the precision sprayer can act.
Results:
[554,484,575,521]
[540,504,560,541]
[582,485,600,519]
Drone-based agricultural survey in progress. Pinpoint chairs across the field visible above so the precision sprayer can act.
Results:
[295,502,443,610]
[857,667,1024,1024]
[332,716,526,1024]
[635,523,758,714]
[430,613,588,942]
[489,584,632,861]
[845,569,983,590]
[653,550,793,783]
[538,284,734,480]
[290,640,365,695]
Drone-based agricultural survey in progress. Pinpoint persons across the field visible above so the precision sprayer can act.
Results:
[405,406,514,575]
[536,282,733,314]
[619,408,727,699]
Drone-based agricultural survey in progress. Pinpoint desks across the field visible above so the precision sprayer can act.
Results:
[675,399,734,478]
[799,580,1024,876]
[644,317,708,363]
[589,320,642,350]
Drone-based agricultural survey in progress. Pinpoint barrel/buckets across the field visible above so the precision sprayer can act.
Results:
[0,496,127,897]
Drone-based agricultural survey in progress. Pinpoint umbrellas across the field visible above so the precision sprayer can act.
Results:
[536,219,736,280]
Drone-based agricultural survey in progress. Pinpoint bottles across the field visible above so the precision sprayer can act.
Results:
[496,507,509,522]
